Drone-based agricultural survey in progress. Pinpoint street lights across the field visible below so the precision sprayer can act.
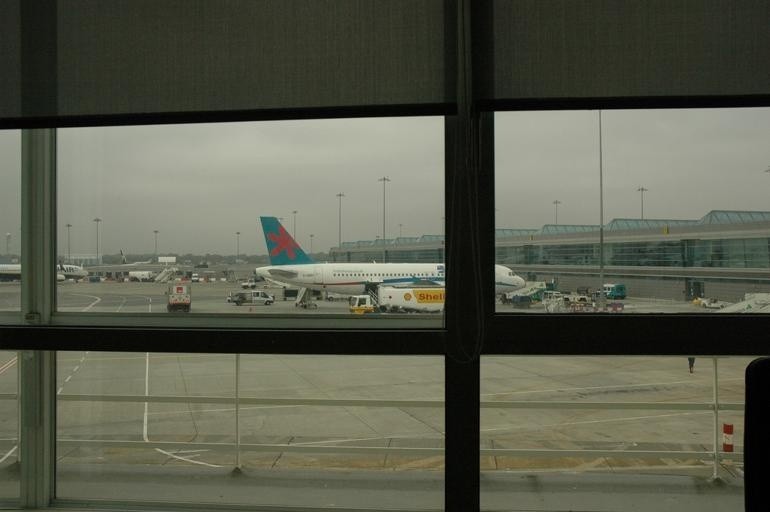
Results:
[637,186,648,219]
[66,224,72,261]
[153,230,159,264]
[552,200,561,224]
[292,210,314,253]
[94,218,100,263]
[4,232,11,259]
[379,177,392,245]
[335,192,345,249]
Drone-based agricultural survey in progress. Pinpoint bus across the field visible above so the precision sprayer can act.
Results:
[597,284,626,299]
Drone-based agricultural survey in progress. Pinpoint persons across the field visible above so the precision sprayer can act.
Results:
[688,357,695,374]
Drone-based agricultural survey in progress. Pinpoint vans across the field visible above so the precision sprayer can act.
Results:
[192,274,199,282]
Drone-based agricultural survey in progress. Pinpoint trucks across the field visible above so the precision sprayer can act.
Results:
[167,283,191,312]
[348,285,445,314]
[511,296,531,310]
[231,291,274,305]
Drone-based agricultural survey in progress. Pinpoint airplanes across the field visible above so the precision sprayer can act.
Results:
[252,217,525,311]
[1,261,85,284]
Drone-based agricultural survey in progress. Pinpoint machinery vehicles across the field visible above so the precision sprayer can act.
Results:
[242,279,256,289]
